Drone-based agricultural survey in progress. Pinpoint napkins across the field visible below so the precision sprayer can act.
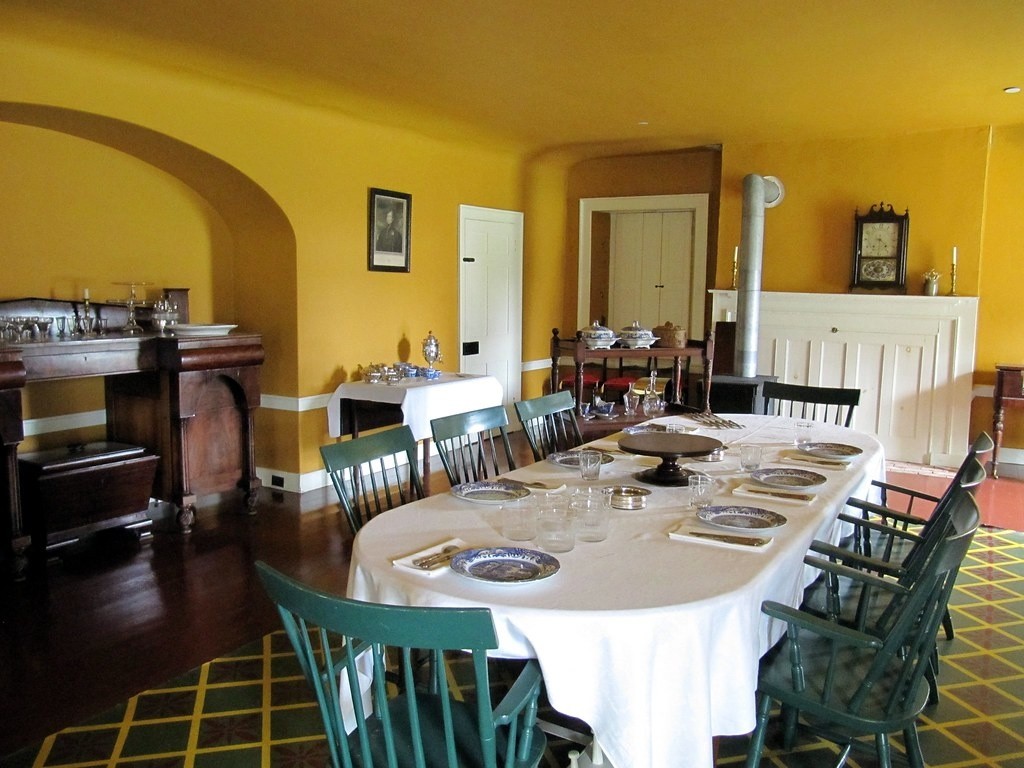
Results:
[393,538,471,579]
[731,483,818,507]
[580,444,633,460]
[669,524,774,553]
[777,452,853,471]
[492,472,567,496]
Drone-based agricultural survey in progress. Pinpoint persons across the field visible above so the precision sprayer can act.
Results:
[375,207,402,253]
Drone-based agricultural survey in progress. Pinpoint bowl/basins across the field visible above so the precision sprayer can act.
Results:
[619,321,660,349]
[580,319,618,349]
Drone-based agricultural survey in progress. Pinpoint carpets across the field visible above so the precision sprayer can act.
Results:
[0,510,1024,768]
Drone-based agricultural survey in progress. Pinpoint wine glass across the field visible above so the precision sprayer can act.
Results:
[0,315,108,344]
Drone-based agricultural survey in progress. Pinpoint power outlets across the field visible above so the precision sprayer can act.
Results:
[272,474,285,488]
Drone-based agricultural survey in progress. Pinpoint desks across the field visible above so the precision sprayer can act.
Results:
[326,373,504,509]
[343,413,884,768]
[991,364,1024,479]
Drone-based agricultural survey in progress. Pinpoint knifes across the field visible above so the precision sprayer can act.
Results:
[421,547,473,570]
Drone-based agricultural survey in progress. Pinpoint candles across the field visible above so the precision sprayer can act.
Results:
[84,289,89,301]
[734,245,738,263]
[952,246,956,264]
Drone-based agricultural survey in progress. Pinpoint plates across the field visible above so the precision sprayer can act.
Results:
[450,482,530,505]
[750,468,827,489]
[696,506,787,531]
[798,442,864,460]
[166,323,238,336]
[546,451,615,468]
[450,548,560,585]
[622,425,679,435]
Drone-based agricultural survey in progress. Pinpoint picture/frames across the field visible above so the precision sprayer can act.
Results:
[368,186,413,273]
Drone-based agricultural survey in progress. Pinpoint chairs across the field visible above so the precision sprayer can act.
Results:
[249,378,1024,768]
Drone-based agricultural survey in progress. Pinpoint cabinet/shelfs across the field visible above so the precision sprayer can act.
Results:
[545,325,719,447]
[708,290,981,468]
[0,297,264,584]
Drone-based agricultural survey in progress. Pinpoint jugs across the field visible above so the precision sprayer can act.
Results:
[642,370,665,417]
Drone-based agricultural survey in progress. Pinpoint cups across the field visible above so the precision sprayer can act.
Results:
[740,444,762,472]
[665,423,685,435]
[579,450,602,480]
[688,475,714,509]
[359,362,441,385]
[794,421,813,445]
[498,489,612,553]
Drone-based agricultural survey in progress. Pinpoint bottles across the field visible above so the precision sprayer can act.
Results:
[623,382,640,414]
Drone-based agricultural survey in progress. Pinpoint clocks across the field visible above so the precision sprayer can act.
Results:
[850,203,911,295]
